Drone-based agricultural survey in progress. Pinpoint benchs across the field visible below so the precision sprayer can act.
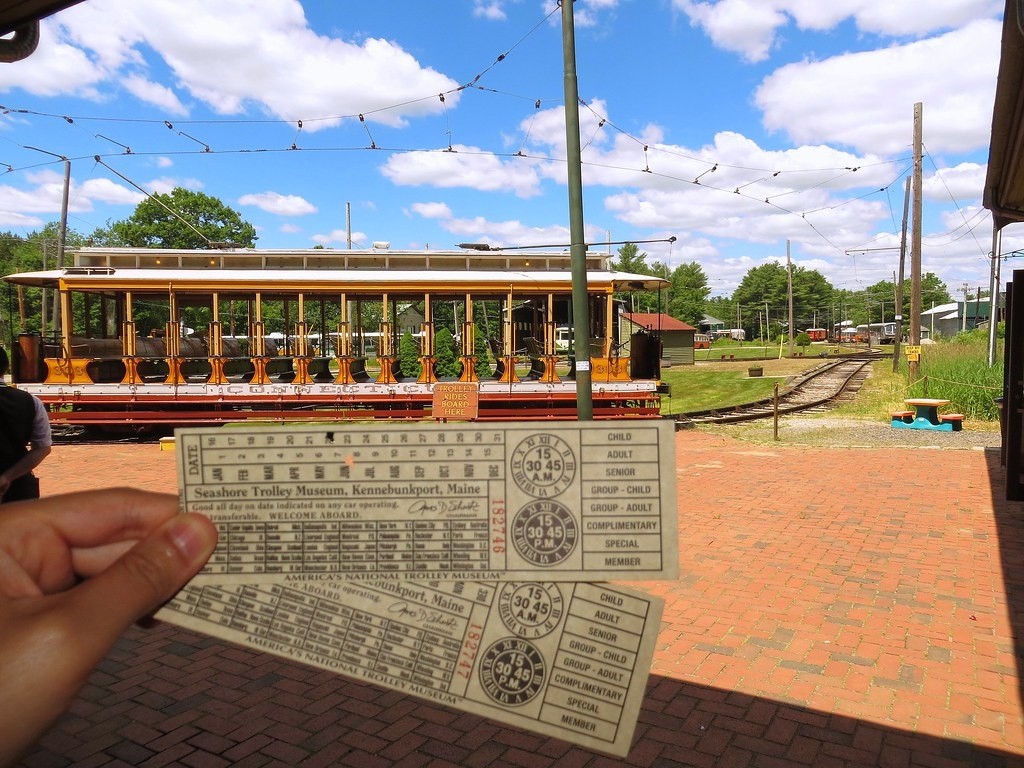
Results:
[939,414,964,431]
[891,411,915,424]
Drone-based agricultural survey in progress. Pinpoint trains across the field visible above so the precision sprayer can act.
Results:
[1,154,678,438]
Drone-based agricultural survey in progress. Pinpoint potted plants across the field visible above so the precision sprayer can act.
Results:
[748,363,763,377]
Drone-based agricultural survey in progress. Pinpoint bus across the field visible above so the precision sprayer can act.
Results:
[694,334,709,349]
[805,322,897,344]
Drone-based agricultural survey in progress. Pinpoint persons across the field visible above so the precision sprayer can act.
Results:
[0,344,53,504]
[0,485,219,768]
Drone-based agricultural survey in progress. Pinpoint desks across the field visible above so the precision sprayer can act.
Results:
[904,399,950,430]
